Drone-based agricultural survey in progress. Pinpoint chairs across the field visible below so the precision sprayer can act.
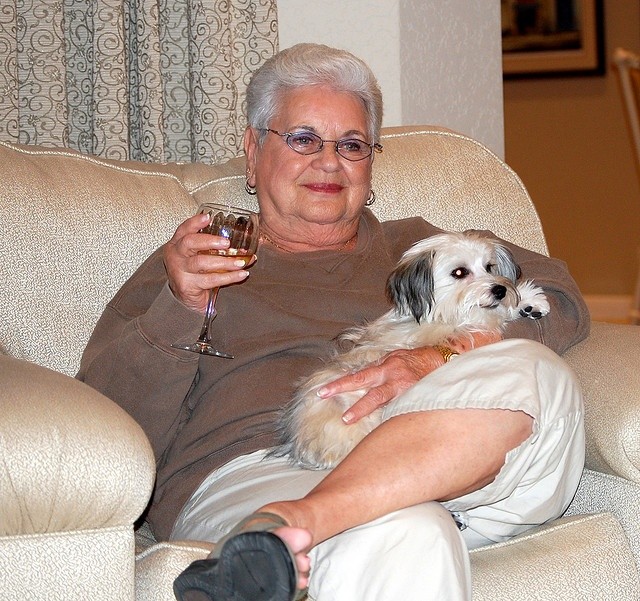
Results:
[610,46,640,325]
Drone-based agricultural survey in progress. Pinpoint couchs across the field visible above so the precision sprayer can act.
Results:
[0,125,640,601]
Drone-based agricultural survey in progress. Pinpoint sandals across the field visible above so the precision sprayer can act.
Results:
[172,512,311,600]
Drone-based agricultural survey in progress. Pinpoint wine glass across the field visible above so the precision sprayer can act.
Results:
[171,201,260,360]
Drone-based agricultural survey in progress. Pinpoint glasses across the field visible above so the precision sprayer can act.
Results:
[258,128,383,161]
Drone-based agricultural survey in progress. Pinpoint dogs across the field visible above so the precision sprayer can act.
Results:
[259,229,551,470]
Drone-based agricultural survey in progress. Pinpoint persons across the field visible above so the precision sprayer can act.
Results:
[75,43,594,601]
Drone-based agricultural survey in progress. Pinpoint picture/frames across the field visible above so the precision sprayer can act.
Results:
[501,0,606,80]
